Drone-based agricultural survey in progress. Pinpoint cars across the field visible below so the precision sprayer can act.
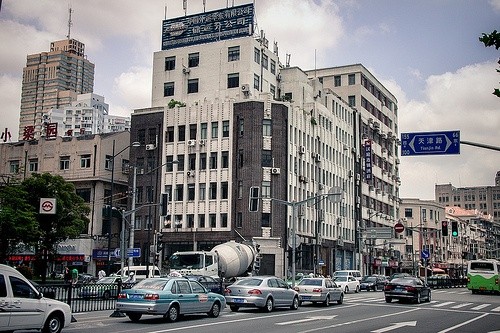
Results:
[79,277,130,300]
[224,276,299,312]
[191,275,225,295]
[384,277,432,304]
[115,277,226,323]
[360,273,409,292]
[294,277,344,306]
[333,276,360,294]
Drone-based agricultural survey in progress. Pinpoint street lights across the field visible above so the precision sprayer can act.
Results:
[106,142,141,274]
[146,160,180,279]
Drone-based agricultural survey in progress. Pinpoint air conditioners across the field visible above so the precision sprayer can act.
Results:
[423,218,428,222]
[187,140,195,147]
[435,219,439,223]
[276,74,281,81]
[299,145,321,184]
[335,239,341,245]
[337,218,342,224]
[300,237,308,244]
[241,84,249,92]
[311,239,317,245]
[146,144,154,150]
[182,68,190,74]
[198,140,205,146]
[186,171,194,177]
[425,237,428,241]
[436,238,439,242]
[344,118,408,243]
[271,168,281,175]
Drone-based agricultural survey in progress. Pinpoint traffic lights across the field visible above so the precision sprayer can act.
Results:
[442,221,448,236]
[452,221,458,236]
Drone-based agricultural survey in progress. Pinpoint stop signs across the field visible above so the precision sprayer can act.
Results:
[394,223,404,233]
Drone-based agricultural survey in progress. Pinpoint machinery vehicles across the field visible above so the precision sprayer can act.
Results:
[164,238,262,286]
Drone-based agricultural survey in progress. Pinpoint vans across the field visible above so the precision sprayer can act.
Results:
[116,266,161,280]
[0,264,72,333]
[334,270,363,282]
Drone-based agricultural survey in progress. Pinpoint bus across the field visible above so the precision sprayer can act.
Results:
[467,258,500,295]
